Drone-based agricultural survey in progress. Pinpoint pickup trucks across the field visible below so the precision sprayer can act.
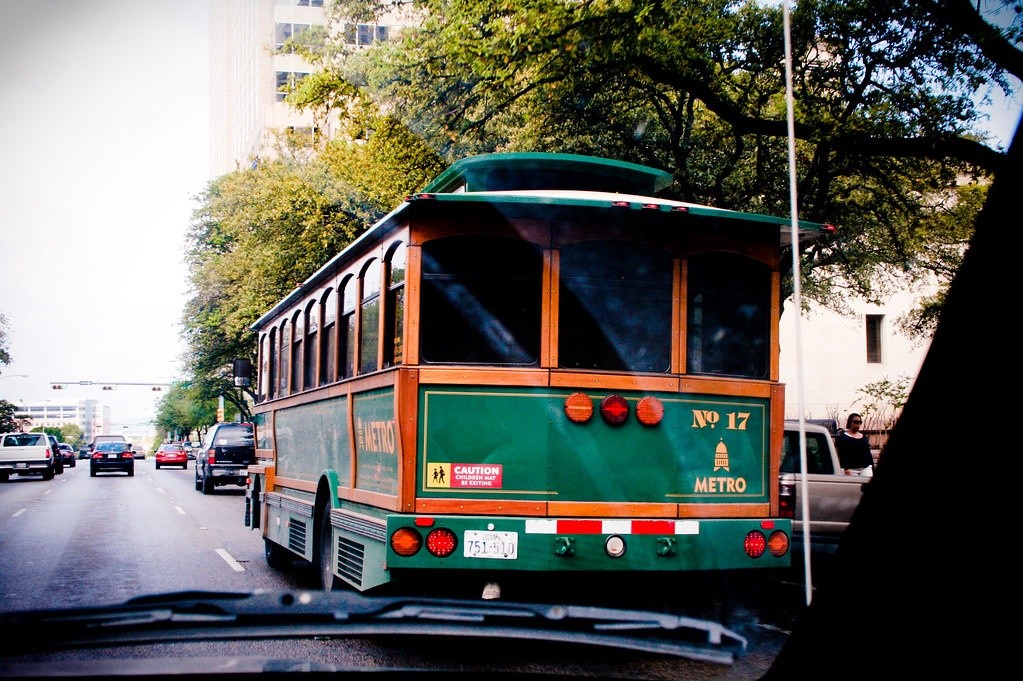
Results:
[780,418,873,543]
[0,430,55,481]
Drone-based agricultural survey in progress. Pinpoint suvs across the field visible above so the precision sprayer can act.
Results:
[17,434,64,476]
[190,420,259,495]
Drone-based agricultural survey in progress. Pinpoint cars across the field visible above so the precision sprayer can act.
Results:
[89,440,134,477]
[165,439,202,460]
[88,435,127,453]
[58,442,76,467]
[130,445,146,459]
[154,443,189,470]
[79,446,91,459]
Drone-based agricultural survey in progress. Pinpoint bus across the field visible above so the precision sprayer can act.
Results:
[233,151,796,592]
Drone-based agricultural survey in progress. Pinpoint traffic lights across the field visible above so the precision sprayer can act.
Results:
[103,386,112,389]
[53,385,63,389]
[152,387,161,391]
